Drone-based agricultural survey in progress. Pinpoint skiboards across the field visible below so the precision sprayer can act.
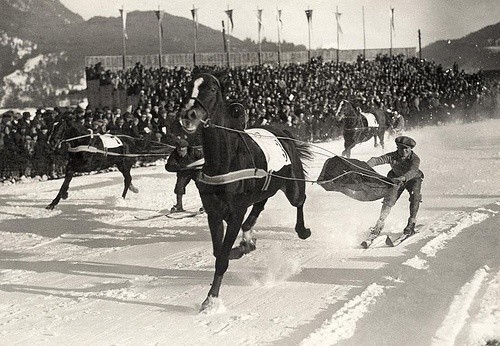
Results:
[134,208,205,221]
[361,222,424,249]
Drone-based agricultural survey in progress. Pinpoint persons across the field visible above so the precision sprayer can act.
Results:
[0,61,163,184]
[389,54,500,107]
[406,108,499,129]
[363,136,425,236]
[164,52,389,142]
[389,108,405,138]
[164,139,205,212]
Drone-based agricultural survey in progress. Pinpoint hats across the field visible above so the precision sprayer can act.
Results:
[175,140,188,147]
[395,136,416,148]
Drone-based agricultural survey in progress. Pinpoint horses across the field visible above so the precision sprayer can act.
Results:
[177,67,316,310]
[334,98,395,159]
[45,117,152,210]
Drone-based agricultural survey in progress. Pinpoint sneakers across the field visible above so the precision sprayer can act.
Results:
[370,225,383,234]
[404,222,415,234]
[171,205,182,212]
